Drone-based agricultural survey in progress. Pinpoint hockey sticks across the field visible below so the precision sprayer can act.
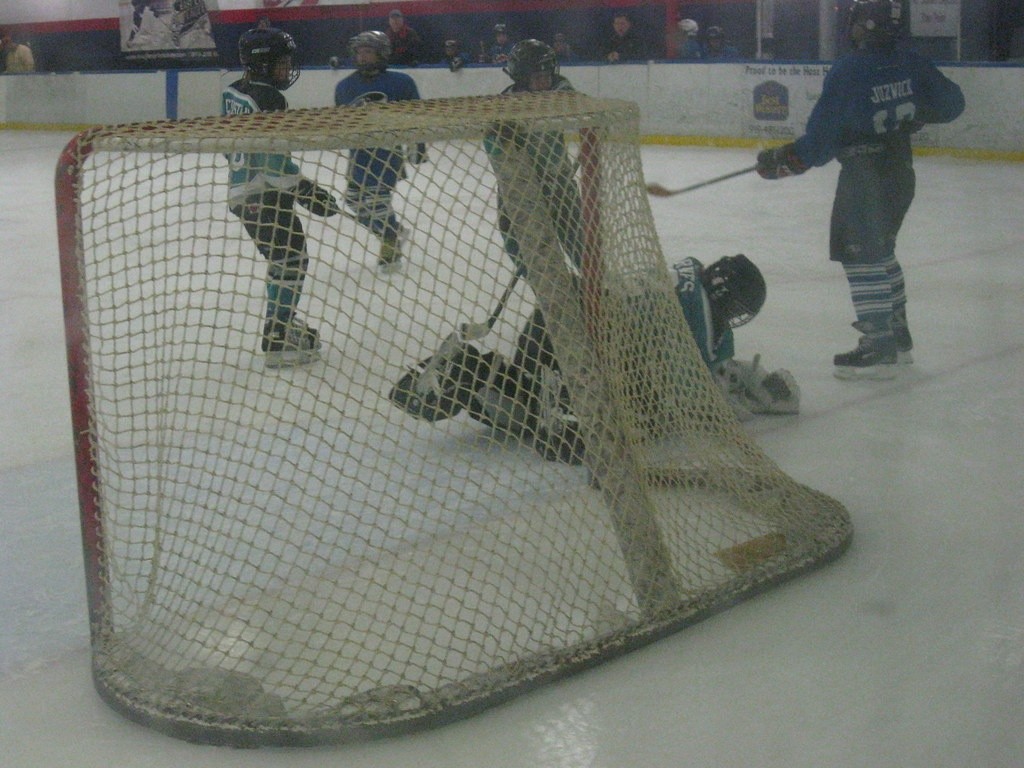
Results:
[460,149,582,341]
[338,208,383,238]
[646,165,758,198]
[585,449,760,492]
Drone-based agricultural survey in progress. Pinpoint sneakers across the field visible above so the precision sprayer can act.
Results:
[377,235,404,274]
[393,221,411,243]
[832,320,898,380]
[851,303,914,364]
[261,311,322,367]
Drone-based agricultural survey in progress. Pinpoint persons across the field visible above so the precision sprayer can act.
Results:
[218,28,338,368]
[390,252,799,466]
[0,29,36,73]
[756,0,965,378]
[383,9,424,66]
[431,30,474,67]
[334,31,429,274]
[319,24,356,66]
[480,38,582,289]
[477,23,514,65]
[646,20,702,58]
[701,26,738,59]
[590,10,648,62]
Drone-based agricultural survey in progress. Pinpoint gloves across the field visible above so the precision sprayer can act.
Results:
[755,143,809,180]
[297,180,338,217]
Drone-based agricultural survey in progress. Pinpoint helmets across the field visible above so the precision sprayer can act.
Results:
[350,31,391,77]
[701,254,767,329]
[680,19,699,36]
[508,40,560,92]
[238,28,301,90]
[706,27,722,40]
[848,0,902,47]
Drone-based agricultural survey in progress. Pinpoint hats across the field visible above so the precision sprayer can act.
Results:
[389,9,402,18]
[445,40,455,48]
[493,24,507,34]
